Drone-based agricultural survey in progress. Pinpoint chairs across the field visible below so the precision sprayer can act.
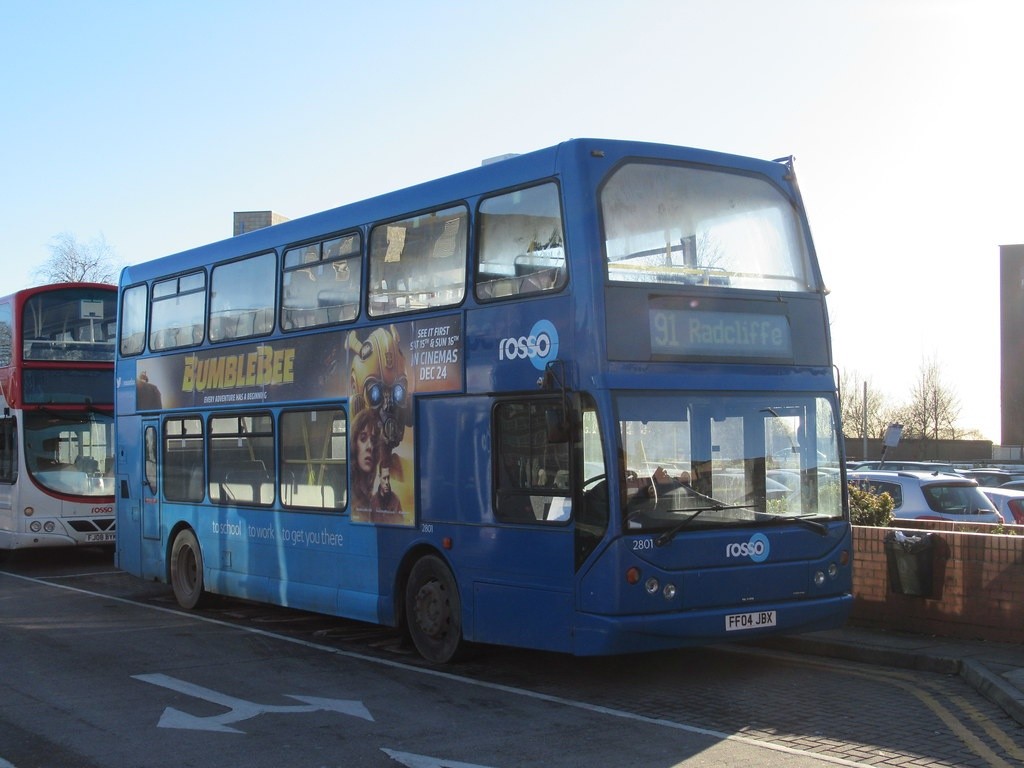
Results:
[215,460,269,487]
[30,337,51,359]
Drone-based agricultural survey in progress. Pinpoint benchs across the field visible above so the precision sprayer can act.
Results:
[475,261,513,283]
[515,255,612,278]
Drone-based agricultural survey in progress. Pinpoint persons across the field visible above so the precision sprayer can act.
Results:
[350,411,403,523]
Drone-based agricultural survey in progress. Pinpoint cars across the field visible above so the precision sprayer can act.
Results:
[585,445,1024,524]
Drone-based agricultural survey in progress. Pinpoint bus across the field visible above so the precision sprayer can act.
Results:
[113,137,856,666]
[1,283,118,549]
